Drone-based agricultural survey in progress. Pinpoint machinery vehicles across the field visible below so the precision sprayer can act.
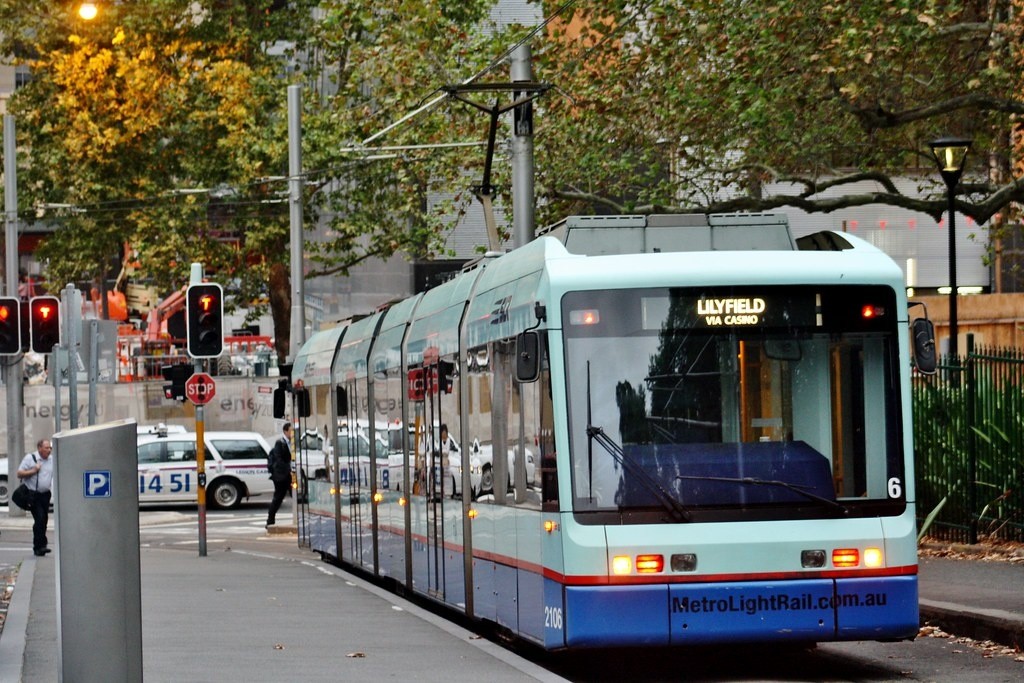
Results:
[90,237,209,382]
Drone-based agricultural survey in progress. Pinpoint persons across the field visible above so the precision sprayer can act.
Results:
[441,425,460,499]
[265,423,292,529]
[322,425,331,481]
[17,440,53,556]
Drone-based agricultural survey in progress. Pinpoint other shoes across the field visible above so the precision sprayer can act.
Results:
[35,547,51,556]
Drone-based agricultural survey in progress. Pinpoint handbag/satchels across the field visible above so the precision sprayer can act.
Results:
[11,484,35,510]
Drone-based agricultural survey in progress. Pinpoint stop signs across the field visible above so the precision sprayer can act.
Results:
[185,372,217,406]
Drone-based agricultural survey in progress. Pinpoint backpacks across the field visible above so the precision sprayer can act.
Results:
[267,438,286,476]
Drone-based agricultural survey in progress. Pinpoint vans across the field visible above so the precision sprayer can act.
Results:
[137,423,276,511]
[297,419,536,500]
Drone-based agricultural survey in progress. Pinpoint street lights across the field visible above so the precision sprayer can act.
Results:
[929,137,970,388]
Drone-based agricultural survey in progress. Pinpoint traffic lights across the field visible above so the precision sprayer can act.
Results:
[162,363,193,403]
[186,282,225,359]
[30,296,62,354]
[0,296,20,356]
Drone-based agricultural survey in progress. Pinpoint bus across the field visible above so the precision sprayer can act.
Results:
[273,212,939,652]
